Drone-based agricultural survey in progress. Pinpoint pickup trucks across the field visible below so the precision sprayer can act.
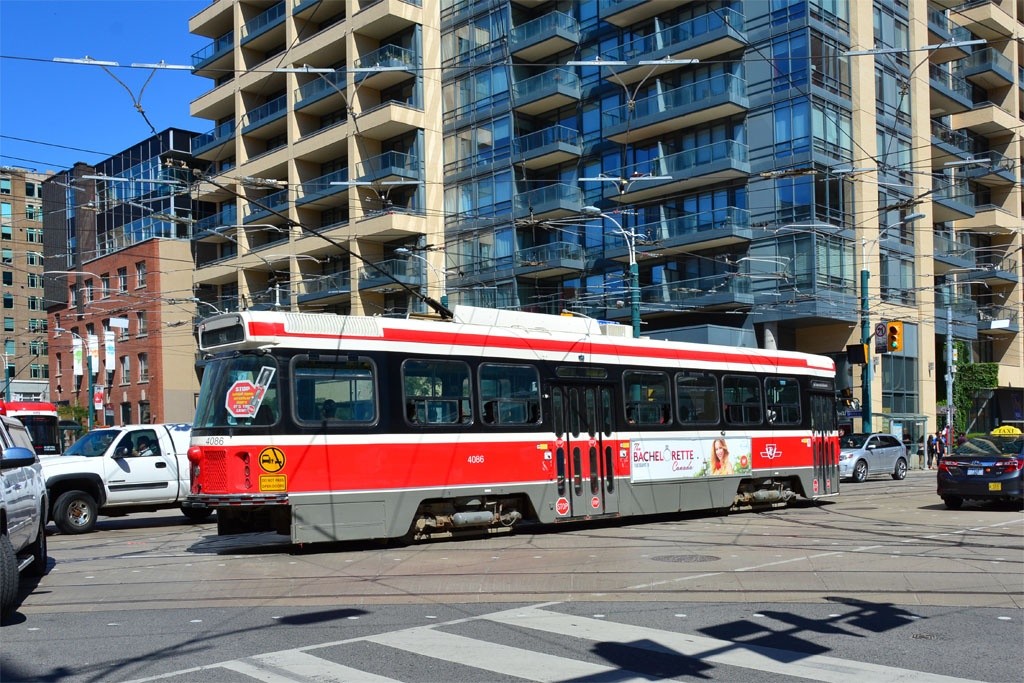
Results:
[40,424,190,535]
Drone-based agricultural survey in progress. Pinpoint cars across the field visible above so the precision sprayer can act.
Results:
[935,433,1023,510]
[840,432,908,481]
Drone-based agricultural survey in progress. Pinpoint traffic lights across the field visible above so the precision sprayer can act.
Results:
[846,343,868,364]
[887,321,904,351]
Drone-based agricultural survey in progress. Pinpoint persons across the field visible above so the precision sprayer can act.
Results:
[405,402,415,422]
[482,401,497,423]
[528,404,540,423]
[936,430,944,466]
[708,439,733,476]
[928,435,934,469]
[626,404,634,423]
[323,399,339,421]
[918,435,924,469]
[679,405,689,421]
[903,428,912,471]
[132,436,153,457]
[959,434,964,446]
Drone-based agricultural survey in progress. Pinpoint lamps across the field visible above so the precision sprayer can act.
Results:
[88,202,94,208]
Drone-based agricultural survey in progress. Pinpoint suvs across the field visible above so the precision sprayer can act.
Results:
[0,413,51,622]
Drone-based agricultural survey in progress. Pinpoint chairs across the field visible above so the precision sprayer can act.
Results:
[251,405,274,425]
[323,400,541,427]
[125,441,133,456]
[149,440,160,455]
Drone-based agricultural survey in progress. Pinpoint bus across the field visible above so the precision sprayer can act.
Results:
[187,178,840,546]
[0,401,61,455]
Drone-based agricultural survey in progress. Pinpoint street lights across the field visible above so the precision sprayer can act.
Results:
[54,327,94,431]
[581,205,641,337]
[395,248,442,319]
[861,211,926,432]
